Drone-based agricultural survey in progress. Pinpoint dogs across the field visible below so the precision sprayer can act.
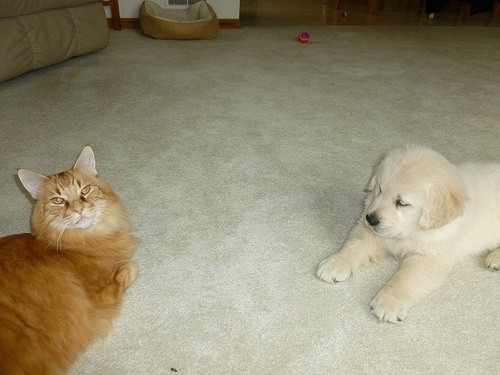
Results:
[313,141,500,326]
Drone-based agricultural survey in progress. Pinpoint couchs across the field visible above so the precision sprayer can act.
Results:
[0,0,111,85]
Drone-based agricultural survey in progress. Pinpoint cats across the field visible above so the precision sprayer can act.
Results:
[0,145,143,375]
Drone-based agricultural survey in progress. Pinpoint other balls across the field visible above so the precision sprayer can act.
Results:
[299,32,310,43]
